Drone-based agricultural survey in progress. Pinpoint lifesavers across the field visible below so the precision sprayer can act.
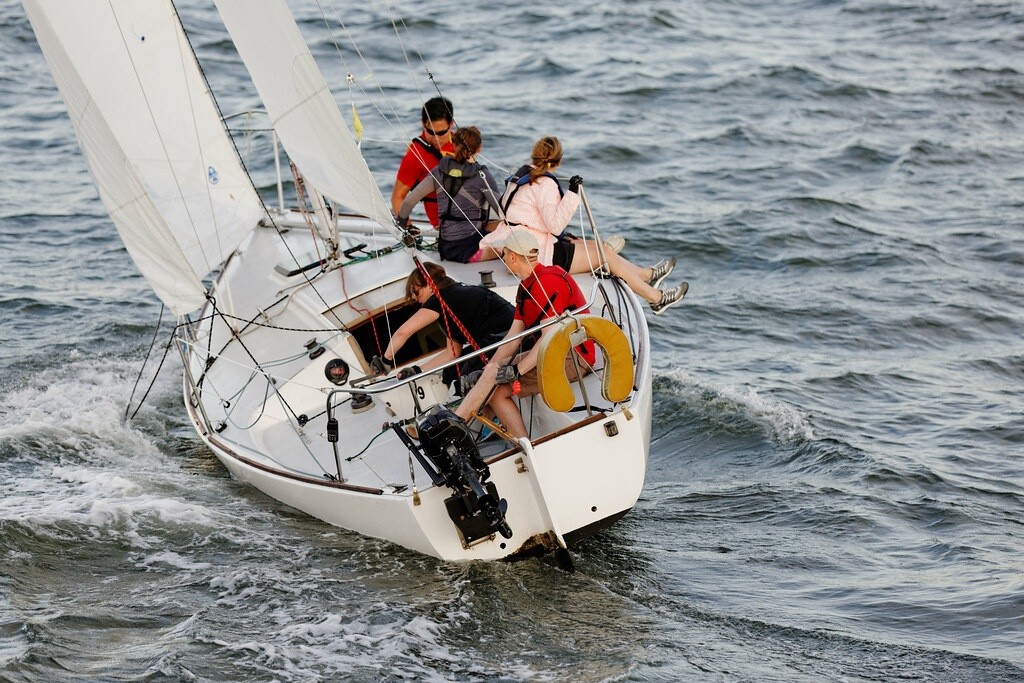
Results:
[535,313,636,413]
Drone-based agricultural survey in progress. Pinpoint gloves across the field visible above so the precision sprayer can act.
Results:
[495,364,517,384]
[567,175,583,193]
[465,370,484,390]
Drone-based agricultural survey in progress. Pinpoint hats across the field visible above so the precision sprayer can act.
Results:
[493,230,539,257]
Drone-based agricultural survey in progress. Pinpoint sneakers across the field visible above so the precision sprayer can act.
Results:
[645,256,677,288]
[651,282,688,315]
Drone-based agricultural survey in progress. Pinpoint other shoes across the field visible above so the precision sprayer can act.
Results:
[604,234,625,254]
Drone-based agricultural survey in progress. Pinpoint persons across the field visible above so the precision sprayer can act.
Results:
[371,261,535,395]
[391,96,457,230]
[479,136,688,315]
[397,126,625,264]
[405,231,595,445]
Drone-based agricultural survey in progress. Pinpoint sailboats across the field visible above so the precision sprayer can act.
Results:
[21,1,650,570]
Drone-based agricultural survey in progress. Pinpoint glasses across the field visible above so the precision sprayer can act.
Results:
[424,124,451,136]
[412,286,423,296]
